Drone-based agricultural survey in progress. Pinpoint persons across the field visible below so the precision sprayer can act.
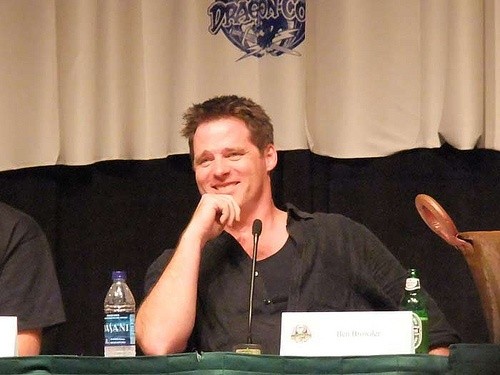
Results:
[135,94,460,357]
[0,198,66,357]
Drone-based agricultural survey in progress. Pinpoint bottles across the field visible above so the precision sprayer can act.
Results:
[400,269,429,354]
[103,271,136,357]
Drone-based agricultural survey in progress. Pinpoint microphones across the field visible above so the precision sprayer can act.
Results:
[234,218,264,354]
